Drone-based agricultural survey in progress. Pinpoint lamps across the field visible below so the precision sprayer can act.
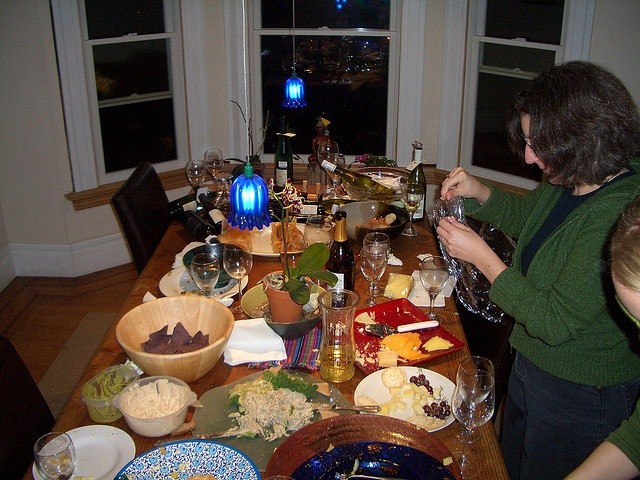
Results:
[227,0,273,232]
[281,0,308,109]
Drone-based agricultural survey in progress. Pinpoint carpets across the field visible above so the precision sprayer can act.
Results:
[37,312,117,421]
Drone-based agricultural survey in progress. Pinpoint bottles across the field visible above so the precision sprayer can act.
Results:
[300,204,324,214]
[301,192,323,201]
[179,210,225,250]
[406,148,427,223]
[317,289,360,384]
[275,116,293,192]
[321,160,395,198]
[198,193,228,234]
[169,191,217,221]
[326,210,356,292]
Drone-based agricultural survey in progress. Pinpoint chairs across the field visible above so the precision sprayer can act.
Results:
[456,221,516,427]
[111,160,176,274]
[0,335,56,480]
[432,214,484,239]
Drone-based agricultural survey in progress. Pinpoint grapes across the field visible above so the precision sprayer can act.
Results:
[409,375,434,395]
[423,401,449,420]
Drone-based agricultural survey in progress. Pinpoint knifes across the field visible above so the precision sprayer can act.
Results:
[319,405,382,413]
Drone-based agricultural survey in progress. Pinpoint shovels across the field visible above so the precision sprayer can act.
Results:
[364,320,439,336]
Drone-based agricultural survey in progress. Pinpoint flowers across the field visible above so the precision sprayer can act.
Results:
[257,177,338,305]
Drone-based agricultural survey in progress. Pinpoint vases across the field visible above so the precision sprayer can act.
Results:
[263,270,312,323]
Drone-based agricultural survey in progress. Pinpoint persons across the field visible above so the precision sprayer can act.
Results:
[560,185,640,480]
[435,58,639,480]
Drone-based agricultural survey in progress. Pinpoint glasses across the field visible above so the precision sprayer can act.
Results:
[523,137,533,146]
[613,294,640,330]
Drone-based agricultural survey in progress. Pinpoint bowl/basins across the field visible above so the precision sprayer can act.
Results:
[81,364,140,424]
[115,296,235,383]
[113,375,203,438]
[263,415,464,480]
[241,283,331,339]
[289,441,457,480]
[183,243,243,288]
[336,201,410,245]
[350,166,412,200]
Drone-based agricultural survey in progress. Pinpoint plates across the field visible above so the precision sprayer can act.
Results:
[113,439,261,480]
[32,424,136,480]
[247,222,332,258]
[317,298,465,376]
[354,365,460,433]
[159,266,249,302]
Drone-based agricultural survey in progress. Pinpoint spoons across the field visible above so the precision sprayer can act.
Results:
[365,320,440,338]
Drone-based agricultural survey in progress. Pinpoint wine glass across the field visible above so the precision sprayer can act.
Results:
[214,172,234,212]
[419,255,450,325]
[359,245,388,306]
[325,153,346,200]
[32,432,76,480]
[186,160,206,188]
[362,232,390,297]
[190,252,220,298]
[400,182,424,237]
[317,141,339,194]
[452,355,496,444]
[222,241,253,316]
[205,148,223,182]
[303,214,334,287]
[451,368,496,479]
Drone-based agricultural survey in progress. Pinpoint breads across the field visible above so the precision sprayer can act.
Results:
[216,225,252,251]
[272,221,305,251]
